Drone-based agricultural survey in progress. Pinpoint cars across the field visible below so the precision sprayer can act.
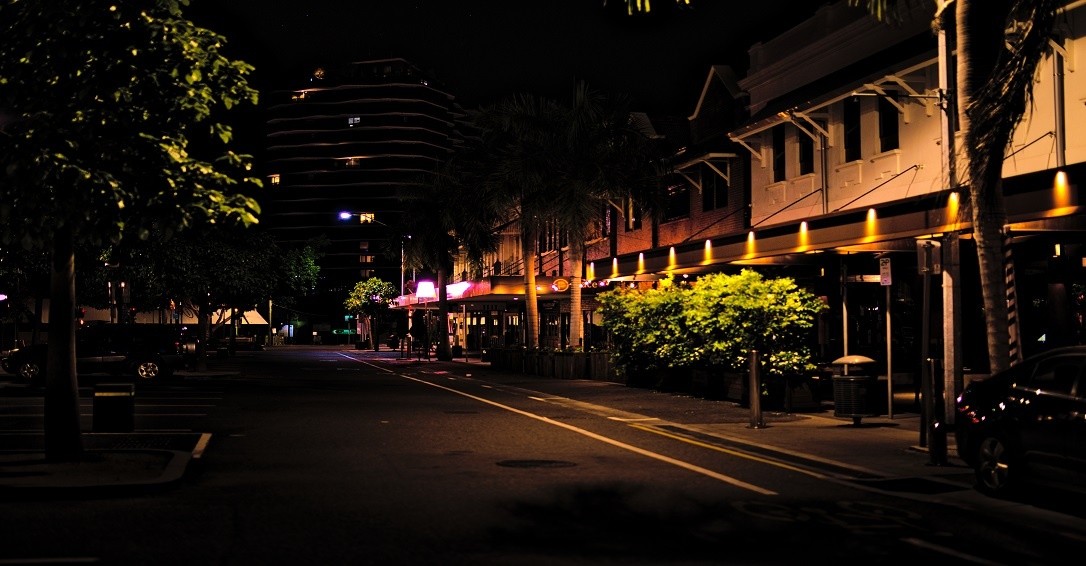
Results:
[956,344,1086,499]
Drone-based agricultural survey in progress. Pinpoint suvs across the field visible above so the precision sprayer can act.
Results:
[2,322,197,385]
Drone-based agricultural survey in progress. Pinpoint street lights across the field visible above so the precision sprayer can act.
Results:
[339,212,412,296]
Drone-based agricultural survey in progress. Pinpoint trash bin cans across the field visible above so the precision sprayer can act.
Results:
[92,383,135,432]
[831,355,880,426]
